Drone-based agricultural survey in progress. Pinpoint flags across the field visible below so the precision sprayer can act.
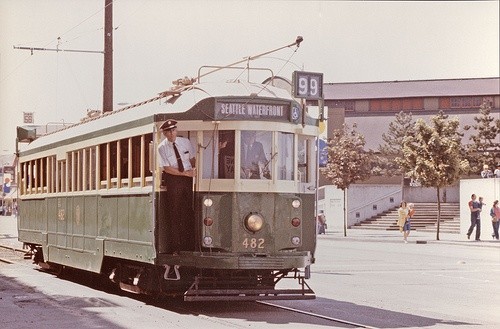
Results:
[3,173,11,193]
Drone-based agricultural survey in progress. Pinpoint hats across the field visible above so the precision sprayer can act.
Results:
[160,119,177,131]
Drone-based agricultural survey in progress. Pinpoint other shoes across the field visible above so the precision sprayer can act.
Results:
[404,239,408,244]
[492,235,494,239]
[466,234,470,240]
[475,238,480,240]
[173,250,179,257]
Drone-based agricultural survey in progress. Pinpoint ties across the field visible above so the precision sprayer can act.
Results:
[173,143,184,172]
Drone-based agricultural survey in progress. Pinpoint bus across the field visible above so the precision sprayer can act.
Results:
[15,34,325,306]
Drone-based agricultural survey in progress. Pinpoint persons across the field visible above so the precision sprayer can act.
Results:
[156,119,197,252]
[398,199,413,243]
[317,209,326,235]
[475,197,487,219]
[238,129,271,179]
[12,198,17,216]
[218,132,234,179]
[466,194,481,240]
[490,199,500,240]
